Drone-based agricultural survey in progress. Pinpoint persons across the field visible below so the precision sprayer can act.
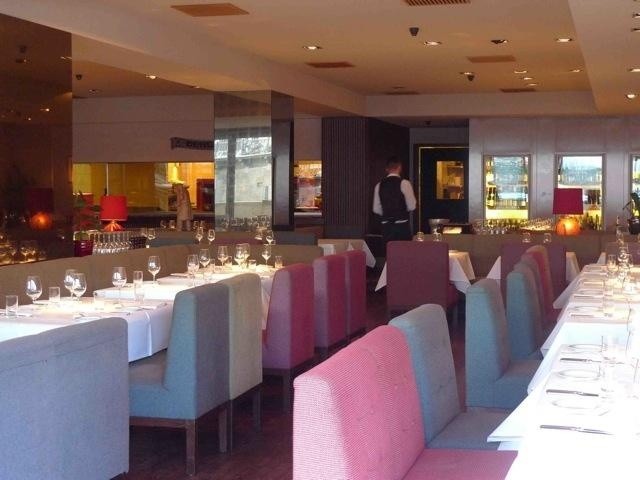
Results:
[172,183,193,233]
[371,156,418,243]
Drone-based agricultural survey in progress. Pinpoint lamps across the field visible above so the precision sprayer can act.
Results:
[99,195,130,232]
[551,185,584,236]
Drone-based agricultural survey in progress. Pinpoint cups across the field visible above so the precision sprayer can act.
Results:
[49,287,60,302]
[433,234,441,240]
[416,231,424,242]
[446,160,464,185]
[5,295,19,316]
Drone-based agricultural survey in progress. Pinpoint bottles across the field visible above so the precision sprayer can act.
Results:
[90,231,116,254]
[314,196,322,207]
[484,158,601,234]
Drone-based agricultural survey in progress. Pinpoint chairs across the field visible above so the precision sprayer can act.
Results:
[1,230,640,480]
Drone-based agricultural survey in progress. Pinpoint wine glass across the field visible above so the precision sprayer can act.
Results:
[64,269,77,309]
[26,275,44,317]
[0,235,40,265]
[73,274,86,316]
[542,226,640,443]
[110,215,287,309]
